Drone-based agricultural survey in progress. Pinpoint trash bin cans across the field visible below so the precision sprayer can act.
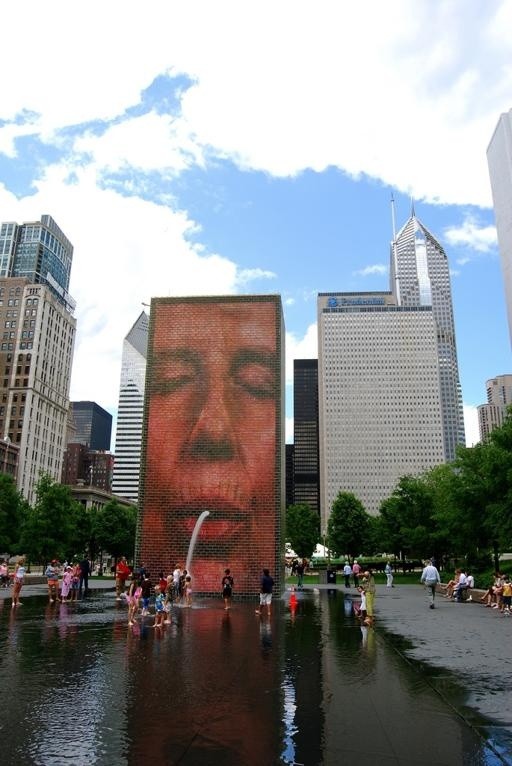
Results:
[318,569,337,584]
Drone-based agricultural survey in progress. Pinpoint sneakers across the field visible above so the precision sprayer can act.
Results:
[440,586,512,617]
[428,602,435,609]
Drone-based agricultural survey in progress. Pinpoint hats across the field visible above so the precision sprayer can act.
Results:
[51,559,72,570]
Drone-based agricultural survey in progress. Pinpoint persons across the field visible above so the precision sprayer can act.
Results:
[421,559,440,608]
[220,569,234,610]
[441,568,475,603]
[256,569,275,615]
[479,570,512,614]
[385,561,394,587]
[0,554,193,628]
[289,557,376,625]
[139,301,278,594]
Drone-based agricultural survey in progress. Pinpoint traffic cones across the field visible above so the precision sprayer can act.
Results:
[287,603,300,627]
[288,584,299,603]
